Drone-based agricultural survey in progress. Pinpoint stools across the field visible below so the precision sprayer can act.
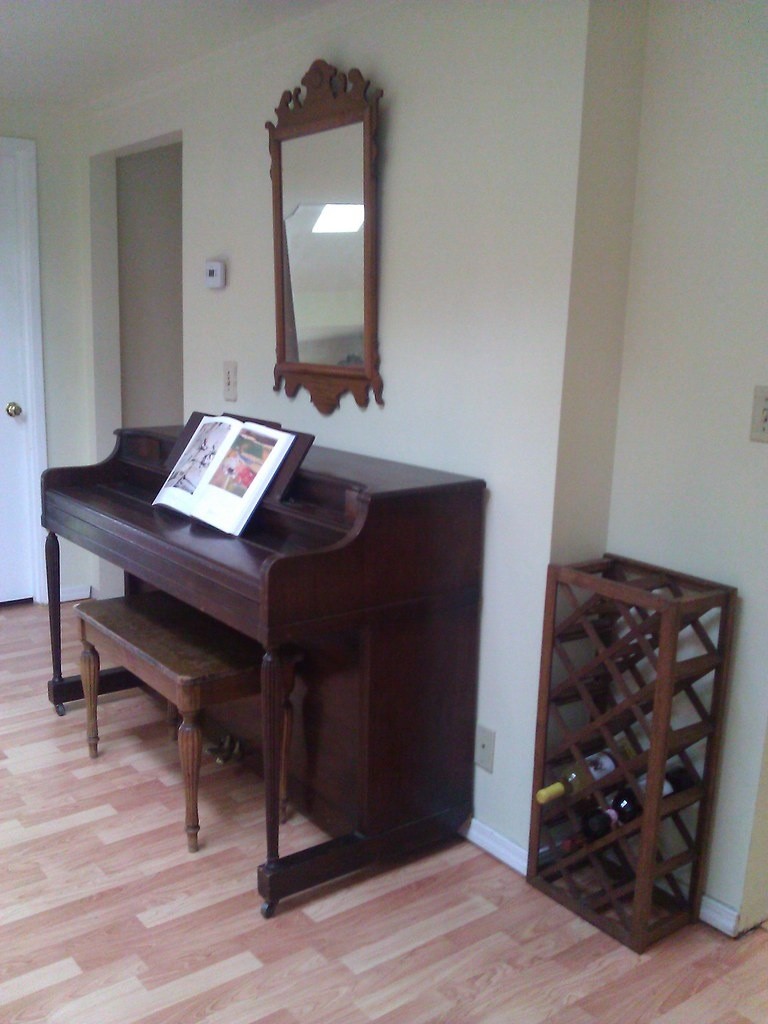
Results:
[75,589,303,855]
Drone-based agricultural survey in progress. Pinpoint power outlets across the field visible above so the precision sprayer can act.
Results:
[221,358,241,405]
[750,385,768,445]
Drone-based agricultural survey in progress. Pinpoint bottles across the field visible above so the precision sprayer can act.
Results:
[535,743,638,806]
[558,808,624,857]
[586,764,694,832]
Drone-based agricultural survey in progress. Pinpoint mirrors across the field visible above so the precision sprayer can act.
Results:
[263,57,387,417]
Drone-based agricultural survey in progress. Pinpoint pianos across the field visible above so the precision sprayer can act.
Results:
[39,410,485,915]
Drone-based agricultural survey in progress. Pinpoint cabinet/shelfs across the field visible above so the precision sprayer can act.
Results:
[525,553,738,955]
